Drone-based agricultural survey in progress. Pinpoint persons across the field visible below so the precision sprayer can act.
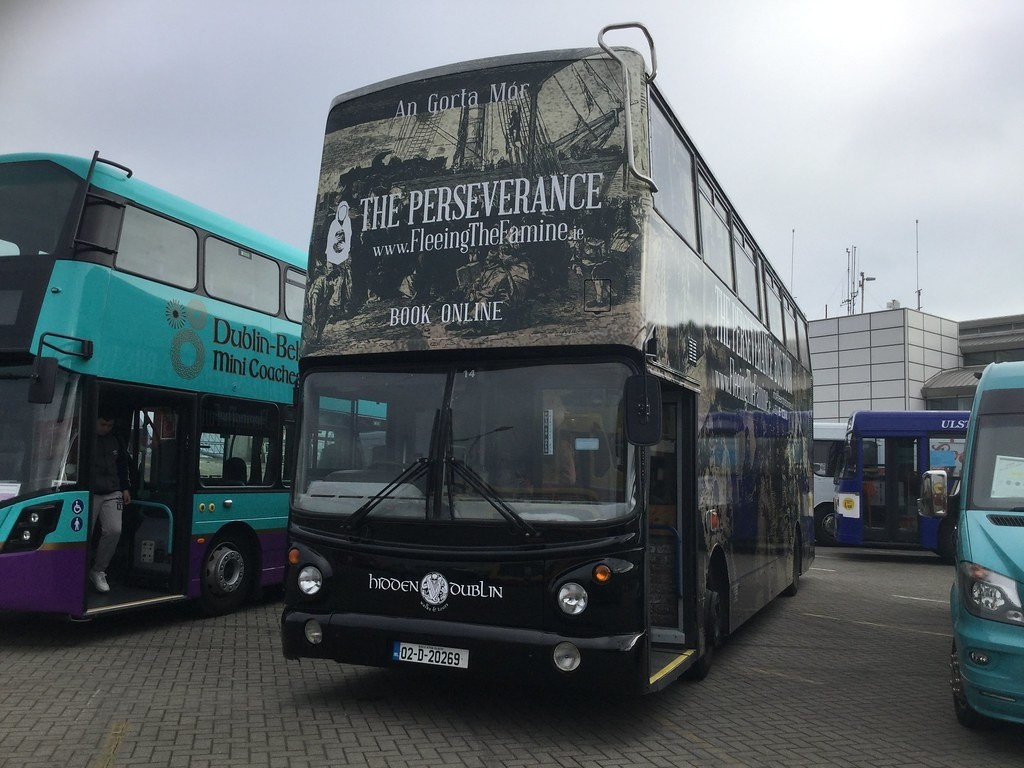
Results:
[65,408,132,593]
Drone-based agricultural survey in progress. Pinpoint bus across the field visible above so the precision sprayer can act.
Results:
[279,20,815,698]
[0,151,391,632]
[831,412,970,561]
[701,410,813,555]
[495,389,676,528]
[813,422,885,549]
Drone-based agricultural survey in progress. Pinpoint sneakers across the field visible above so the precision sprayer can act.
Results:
[90,568,110,592]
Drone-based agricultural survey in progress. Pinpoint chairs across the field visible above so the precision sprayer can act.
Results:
[222,458,248,486]
[318,443,342,469]
[372,446,386,463]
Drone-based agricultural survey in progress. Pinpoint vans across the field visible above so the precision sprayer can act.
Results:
[915,359,1024,728]
